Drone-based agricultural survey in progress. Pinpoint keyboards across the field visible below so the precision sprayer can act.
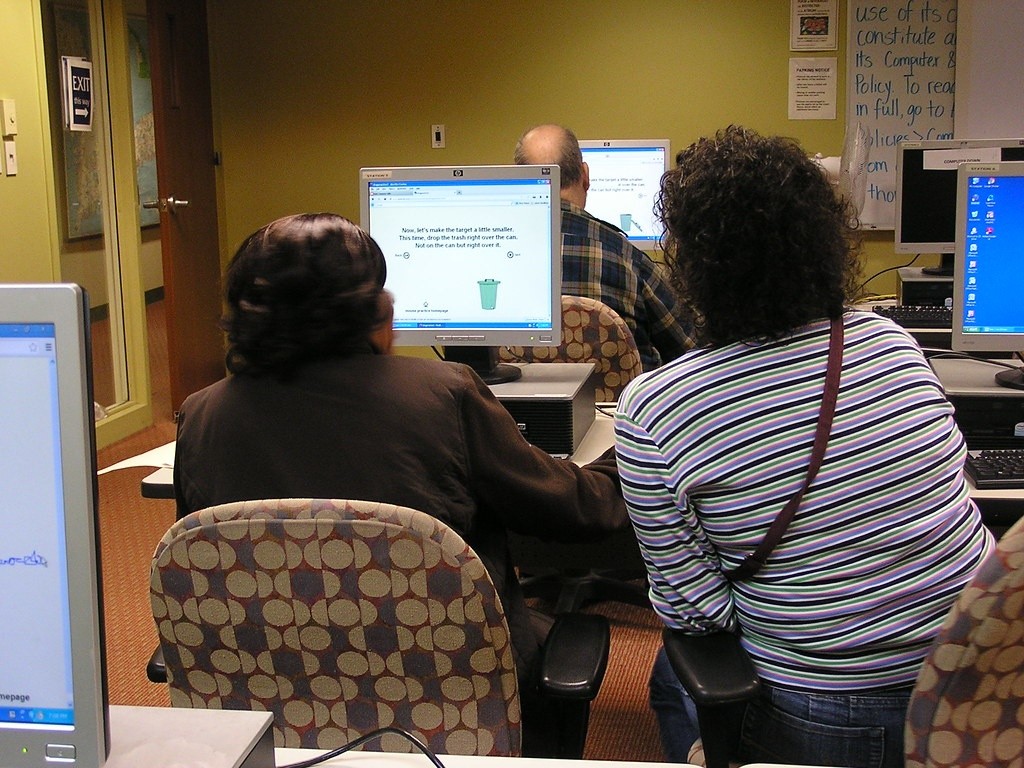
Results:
[962,450,1024,489]
[872,305,953,329]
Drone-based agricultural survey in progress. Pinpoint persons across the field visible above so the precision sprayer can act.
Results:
[615,125,998,768]
[173,212,629,706]
[513,124,712,374]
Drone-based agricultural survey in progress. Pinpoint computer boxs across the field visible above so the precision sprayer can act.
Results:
[487,363,596,458]
[104,706,276,768]
[895,267,954,308]
[928,358,1024,451]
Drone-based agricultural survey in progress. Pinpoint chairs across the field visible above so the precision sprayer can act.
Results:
[147,499,614,768]
[495,294,642,403]
[660,510,1024,768]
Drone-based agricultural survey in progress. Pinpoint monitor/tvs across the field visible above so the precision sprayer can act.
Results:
[949,162,1024,391]
[577,139,670,251]
[0,283,109,768]
[358,165,560,385]
[894,136,1024,278]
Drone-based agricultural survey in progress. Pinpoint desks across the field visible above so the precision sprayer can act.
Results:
[141,298,1024,506]
[104,702,276,768]
